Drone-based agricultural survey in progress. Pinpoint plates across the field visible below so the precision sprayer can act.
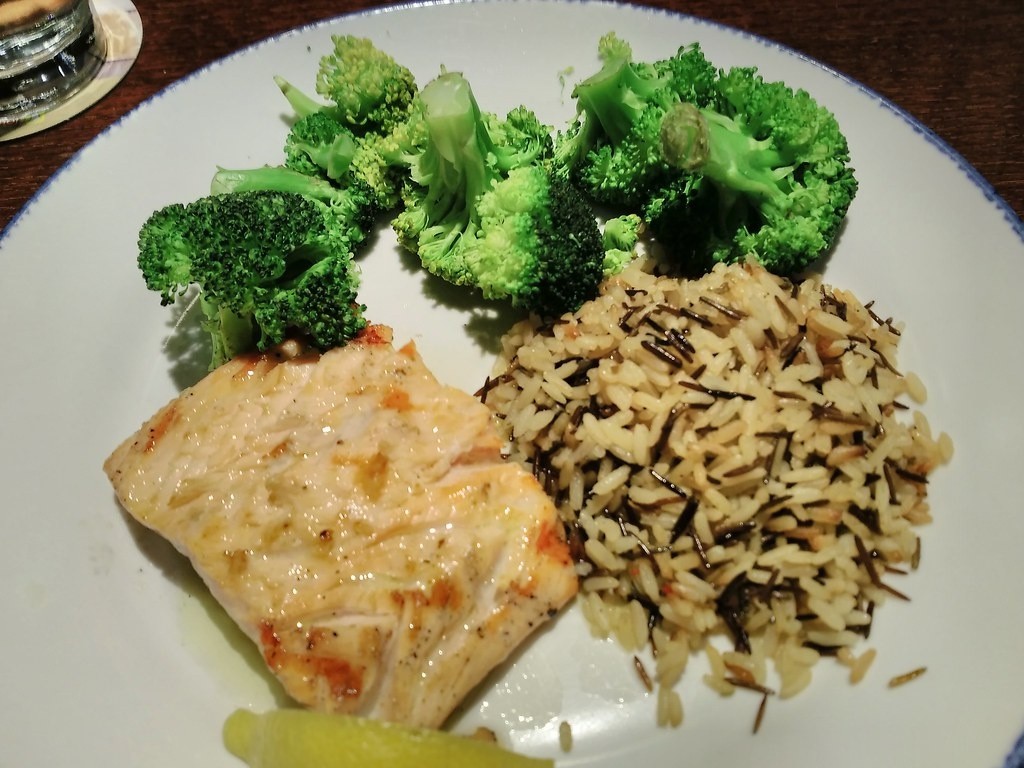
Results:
[0,0,1024,768]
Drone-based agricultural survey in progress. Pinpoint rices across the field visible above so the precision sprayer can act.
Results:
[476,255,954,748]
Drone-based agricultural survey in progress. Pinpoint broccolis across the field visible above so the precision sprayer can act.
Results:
[134,33,861,370]
